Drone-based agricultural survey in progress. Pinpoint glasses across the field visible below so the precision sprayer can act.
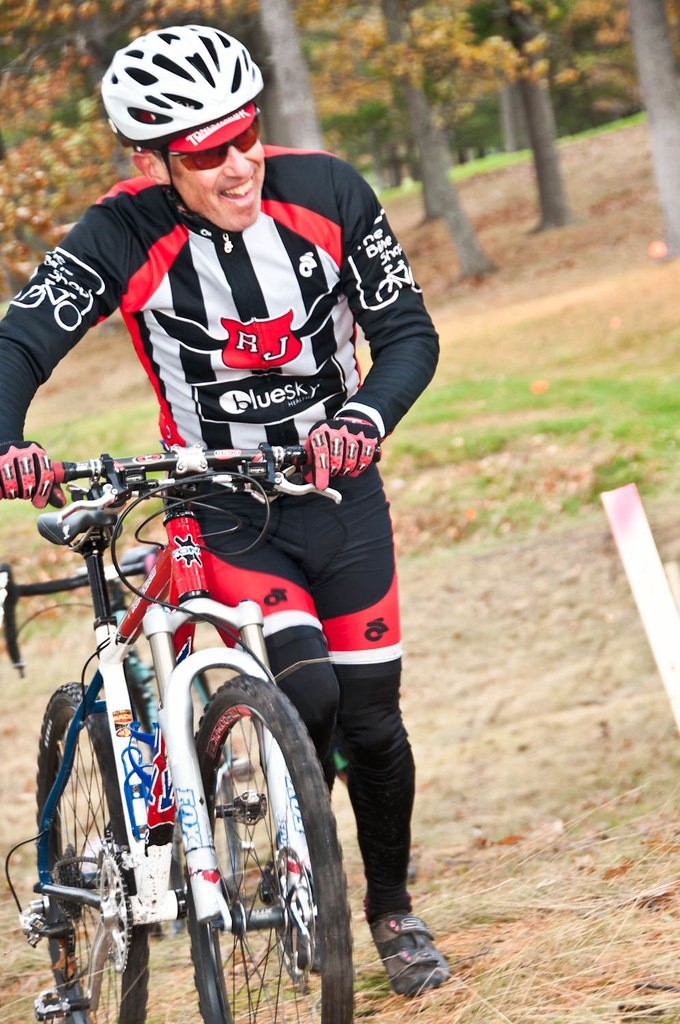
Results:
[158,103,260,172]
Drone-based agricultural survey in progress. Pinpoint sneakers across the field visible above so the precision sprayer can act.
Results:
[371,912,447,997]
[259,878,322,973]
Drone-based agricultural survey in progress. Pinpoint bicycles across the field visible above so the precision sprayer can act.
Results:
[0,440,389,1024]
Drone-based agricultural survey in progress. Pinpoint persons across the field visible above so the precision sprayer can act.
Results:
[0,25,449,999]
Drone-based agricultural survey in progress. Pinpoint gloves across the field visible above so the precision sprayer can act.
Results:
[0,441,66,509]
[300,417,379,491]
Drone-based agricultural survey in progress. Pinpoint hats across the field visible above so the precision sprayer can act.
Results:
[167,105,255,152]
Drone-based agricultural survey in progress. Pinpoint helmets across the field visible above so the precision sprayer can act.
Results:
[100,25,266,150]
